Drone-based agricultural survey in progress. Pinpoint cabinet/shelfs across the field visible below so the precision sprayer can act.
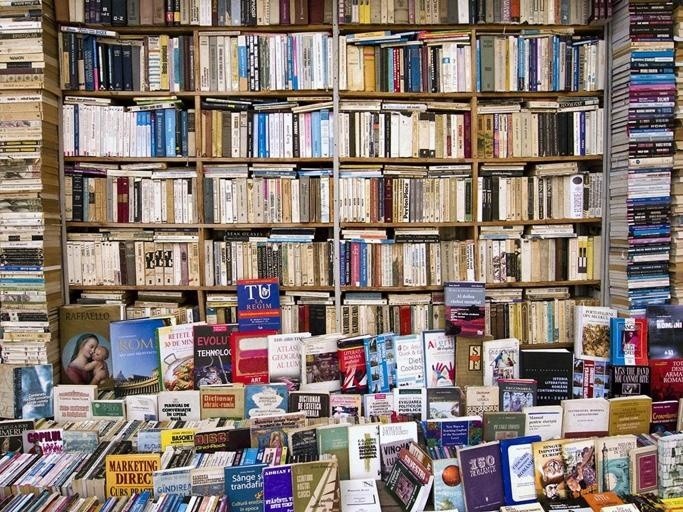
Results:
[335,0,612,350]
[54,0,335,340]
